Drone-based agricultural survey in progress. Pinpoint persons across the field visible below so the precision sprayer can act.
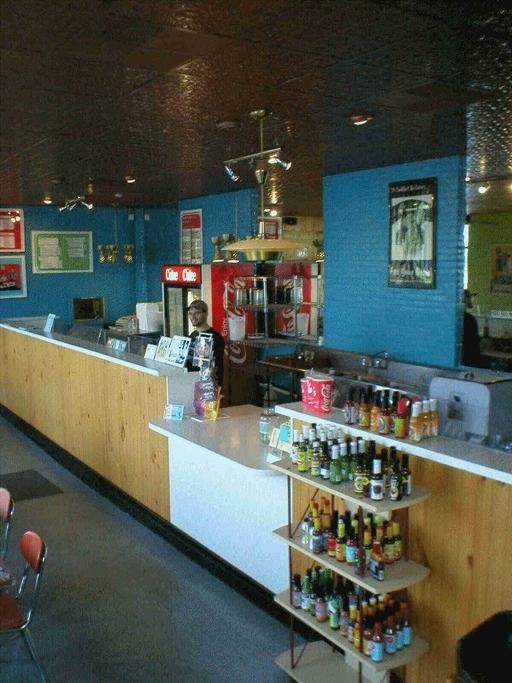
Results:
[463,289,481,368]
[0,267,17,286]
[183,299,224,389]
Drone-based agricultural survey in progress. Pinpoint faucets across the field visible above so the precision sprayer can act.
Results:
[369,351,391,368]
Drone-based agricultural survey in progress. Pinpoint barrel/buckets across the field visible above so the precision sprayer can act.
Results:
[228,316,246,341]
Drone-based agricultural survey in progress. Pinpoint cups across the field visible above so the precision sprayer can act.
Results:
[299,375,335,414]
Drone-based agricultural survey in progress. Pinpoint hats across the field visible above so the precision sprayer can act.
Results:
[186,300,208,312]
[465,289,478,297]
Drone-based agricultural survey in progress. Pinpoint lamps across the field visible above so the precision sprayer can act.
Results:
[58,196,94,212]
[219,107,306,250]
[223,147,291,184]
[96,202,136,264]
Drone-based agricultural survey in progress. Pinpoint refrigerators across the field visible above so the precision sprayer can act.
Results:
[161,262,258,406]
[265,262,319,343]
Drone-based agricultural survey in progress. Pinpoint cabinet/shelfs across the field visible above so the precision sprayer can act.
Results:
[269,458,431,683]
[236,274,319,408]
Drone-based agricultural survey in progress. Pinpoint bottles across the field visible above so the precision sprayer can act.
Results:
[340,387,439,443]
[258,407,280,445]
[290,422,415,501]
[299,496,403,582]
[292,564,413,664]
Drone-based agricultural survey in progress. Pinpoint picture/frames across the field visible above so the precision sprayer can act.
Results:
[0,255,28,299]
[0,208,26,253]
[31,230,93,272]
[388,177,437,290]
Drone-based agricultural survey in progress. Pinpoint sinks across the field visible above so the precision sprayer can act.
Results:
[352,375,374,383]
[377,380,409,389]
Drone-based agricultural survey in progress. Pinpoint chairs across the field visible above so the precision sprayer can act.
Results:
[0,487,19,594]
[0,530,48,682]
